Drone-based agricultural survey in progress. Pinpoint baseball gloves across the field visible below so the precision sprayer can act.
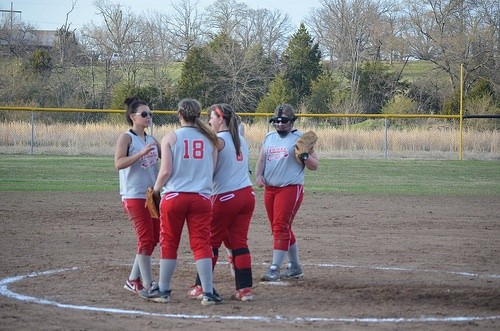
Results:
[294,131,318,165]
[146,187,160,219]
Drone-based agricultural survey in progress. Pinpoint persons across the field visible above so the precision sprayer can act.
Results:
[255,104,320,282]
[115,95,161,295]
[188,103,254,303]
[138,98,223,306]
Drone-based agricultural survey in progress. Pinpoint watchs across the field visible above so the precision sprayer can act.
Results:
[303,154,308,160]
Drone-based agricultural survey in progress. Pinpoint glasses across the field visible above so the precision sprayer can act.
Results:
[272,117,293,124]
[133,111,153,118]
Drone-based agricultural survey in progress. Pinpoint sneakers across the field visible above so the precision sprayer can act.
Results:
[186,284,203,300]
[138,280,172,303]
[124,277,145,297]
[279,261,304,280]
[200,287,224,306]
[260,264,281,282]
[230,287,253,302]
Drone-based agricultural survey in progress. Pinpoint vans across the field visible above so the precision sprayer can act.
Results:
[98,53,121,62]
